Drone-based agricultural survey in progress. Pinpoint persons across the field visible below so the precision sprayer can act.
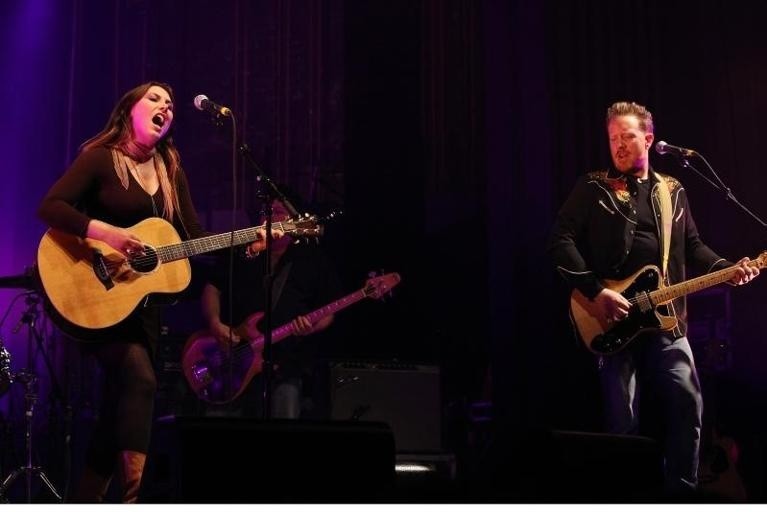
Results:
[543,99,759,503]
[36,77,287,503]
[199,192,339,500]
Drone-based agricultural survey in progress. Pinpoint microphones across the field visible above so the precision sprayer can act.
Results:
[194,94,231,120]
[654,141,695,159]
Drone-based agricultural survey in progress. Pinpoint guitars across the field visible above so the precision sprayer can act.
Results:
[184,270,401,406]
[36,207,324,338]
[568,249,767,355]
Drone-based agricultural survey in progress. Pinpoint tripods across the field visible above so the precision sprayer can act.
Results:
[0,265,65,503]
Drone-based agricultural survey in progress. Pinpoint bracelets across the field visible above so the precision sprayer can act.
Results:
[244,243,262,259]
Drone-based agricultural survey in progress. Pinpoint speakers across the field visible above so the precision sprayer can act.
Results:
[323,359,443,453]
[181,417,394,502]
[525,429,663,504]
